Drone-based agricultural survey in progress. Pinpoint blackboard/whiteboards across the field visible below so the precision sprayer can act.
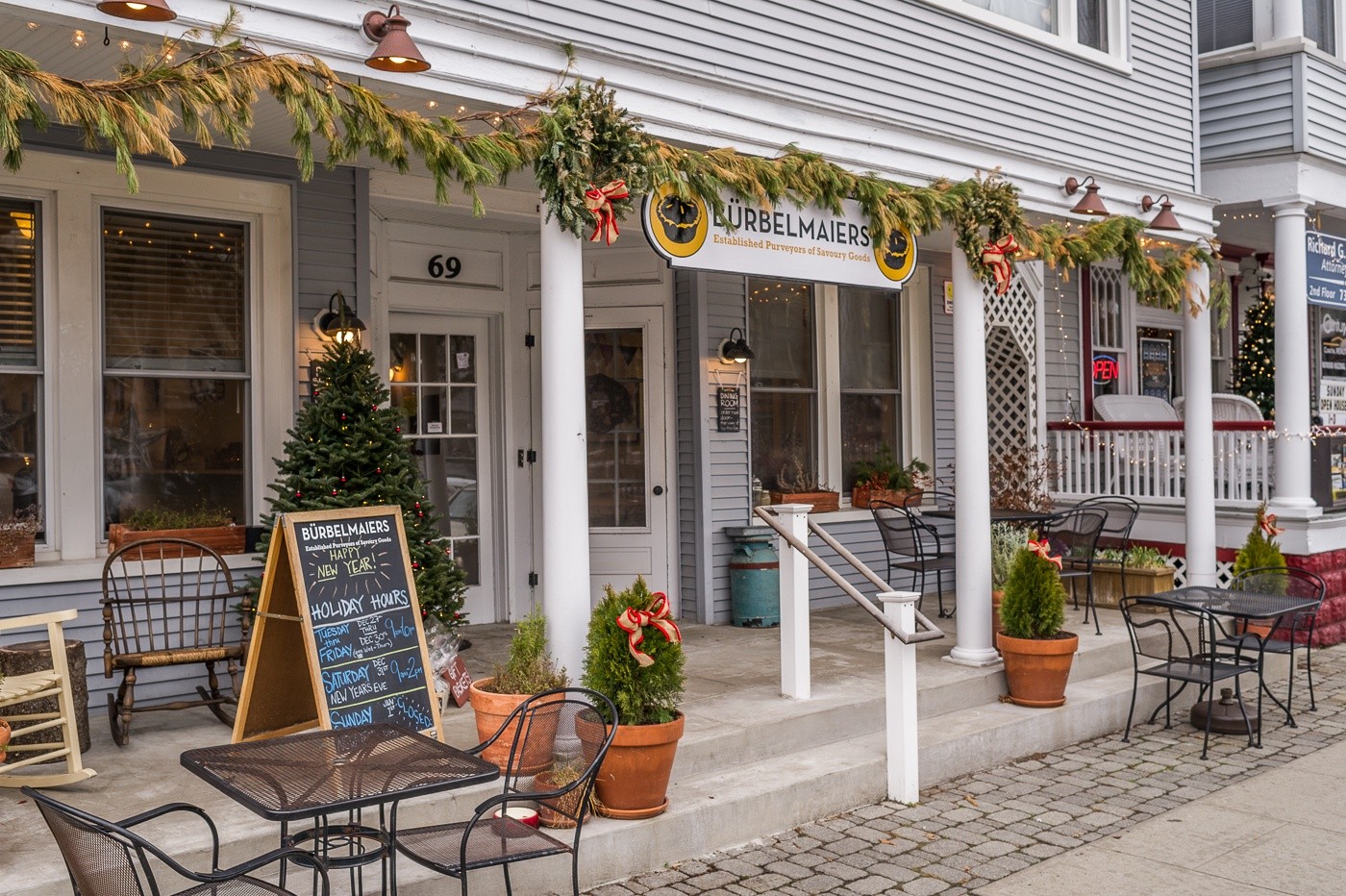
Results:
[222,505,446,803]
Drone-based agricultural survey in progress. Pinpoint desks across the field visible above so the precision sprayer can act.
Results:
[920,507,1064,543]
[179,720,502,896]
[1136,585,1323,749]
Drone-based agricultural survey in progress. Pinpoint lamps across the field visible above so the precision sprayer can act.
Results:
[1064,175,1111,217]
[311,289,367,346]
[389,332,405,373]
[361,4,431,73]
[717,327,757,365]
[95,0,177,22]
[1141,194,1184,232]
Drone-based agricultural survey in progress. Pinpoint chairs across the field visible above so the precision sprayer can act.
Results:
[1028,506,1109,636]
[867,498,956,619]
[97,536,260,745]
[1173,393,1277,500]
[0,608,97,790]
[1015,495,1140,624]
[1091,395,1186,496]
[903,491,957,617]
[1197,565,1328,749]
[1117,594,1300,761]
[378,687,619,896]
[19,783,332,896]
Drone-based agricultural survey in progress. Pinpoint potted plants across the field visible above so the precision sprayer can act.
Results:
[0,502,45,569]
[1055,542,1176,615]
[572,572,688,821]
[769,462,840,515]
[469,604,573,777]
[851,439,930,509]
[997,541,1081,709]
[107,495,247,562]
[1228,496,1291,641]
[534,750,605,829]
[988,521,1040,658]
